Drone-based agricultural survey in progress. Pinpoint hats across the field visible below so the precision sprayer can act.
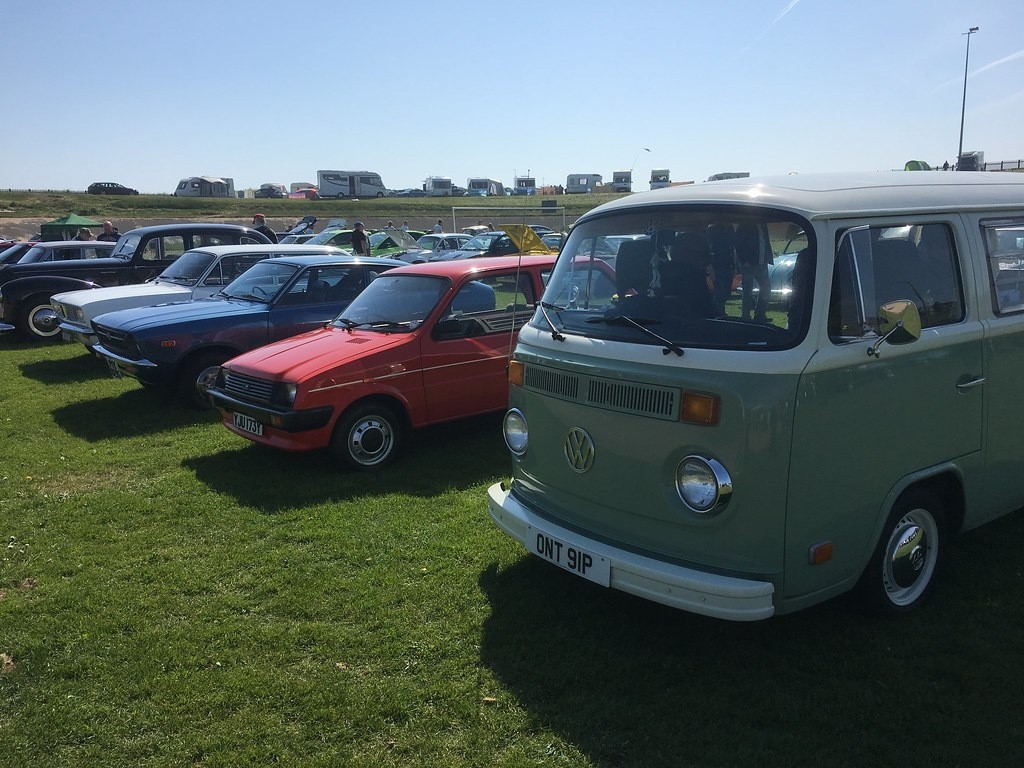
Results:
[80,228,94,236]
[253,214,265,224]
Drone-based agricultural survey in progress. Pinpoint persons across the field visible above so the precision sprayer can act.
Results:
[478,220,483,225]
[95,221,123,258]
[401,221,409,230]
[306,225,315,234]
[68,228,94,259]
[286,225,292,232]
[488,223,495,232]
[352,221,371,256]
[253,214,279,243]
[383,220,395,229]
[679,222,775,324]
[434,219,443,234]
[550,184,563,195]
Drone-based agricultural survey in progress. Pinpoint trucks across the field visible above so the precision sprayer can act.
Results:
[703,171,751,182]
[957,149,986,171]
[648,169,672,190]
[565,173,604,194]
[174,176,235,198]
[610,171,634,193]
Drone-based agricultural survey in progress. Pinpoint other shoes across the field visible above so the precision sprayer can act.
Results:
[754,312,773,322]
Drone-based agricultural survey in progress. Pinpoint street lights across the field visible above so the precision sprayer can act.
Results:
[955,26,980,172]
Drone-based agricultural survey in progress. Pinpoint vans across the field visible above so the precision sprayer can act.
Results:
[481,164,1024,626]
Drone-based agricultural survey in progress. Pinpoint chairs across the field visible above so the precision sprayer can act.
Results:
[870,240,932,333]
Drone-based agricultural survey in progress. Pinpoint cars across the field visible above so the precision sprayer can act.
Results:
[204,253,616,473]
[253,188,284,199]
[737,229,810,305]
[0,222,287,344]
[0,237,137,264]
[48,241,382,358]
[88,254,497,412]
[87,181,133,195]
[206,218,654,298]
[295,188,321,200]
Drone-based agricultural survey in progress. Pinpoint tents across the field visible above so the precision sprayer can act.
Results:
[40,212,103,241]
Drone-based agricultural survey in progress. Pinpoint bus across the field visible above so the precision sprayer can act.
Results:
[513,177,537,195]
[315,170,387,199]
[425,176,452,197]
[467,178,503,197]
